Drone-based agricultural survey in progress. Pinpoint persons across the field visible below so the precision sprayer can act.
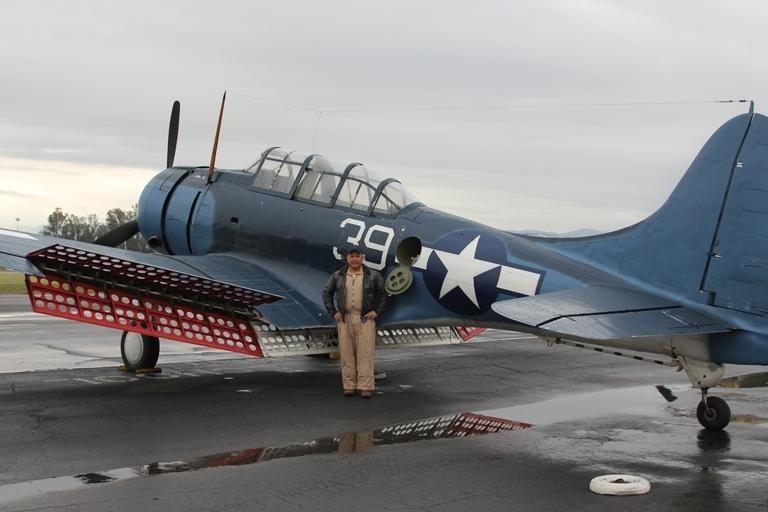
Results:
[322,245,386,398]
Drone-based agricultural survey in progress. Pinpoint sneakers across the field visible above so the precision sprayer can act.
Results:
[361,389,372,398]
[344,389,355,396]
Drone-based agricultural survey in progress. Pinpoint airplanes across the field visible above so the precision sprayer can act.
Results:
[0,91,768,430]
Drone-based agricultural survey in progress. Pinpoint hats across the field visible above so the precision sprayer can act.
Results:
[347,245,363,254]
